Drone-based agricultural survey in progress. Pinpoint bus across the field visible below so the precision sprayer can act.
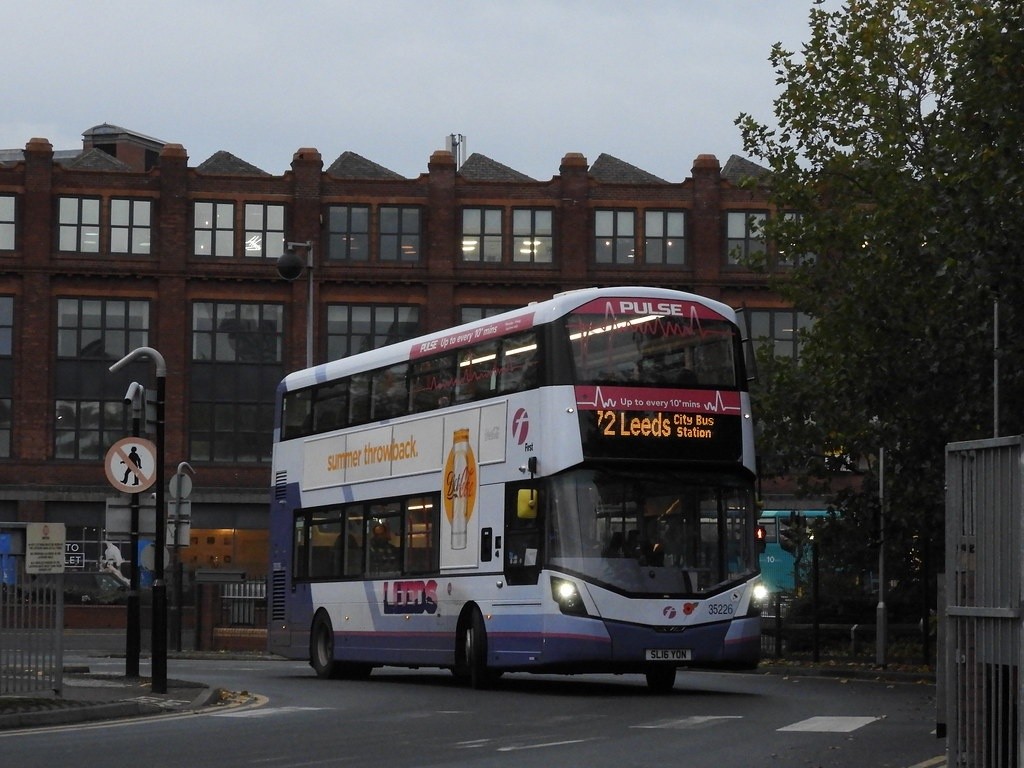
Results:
[265,285,765,696]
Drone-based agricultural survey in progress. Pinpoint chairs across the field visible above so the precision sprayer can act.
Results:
[303,323,704,436]
[330,533,400,574]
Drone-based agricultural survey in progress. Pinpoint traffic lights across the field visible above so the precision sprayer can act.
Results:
[755,525,766,554]
[781,511,799,554]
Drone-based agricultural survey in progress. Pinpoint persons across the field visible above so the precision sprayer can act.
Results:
[372,525,401,570]
